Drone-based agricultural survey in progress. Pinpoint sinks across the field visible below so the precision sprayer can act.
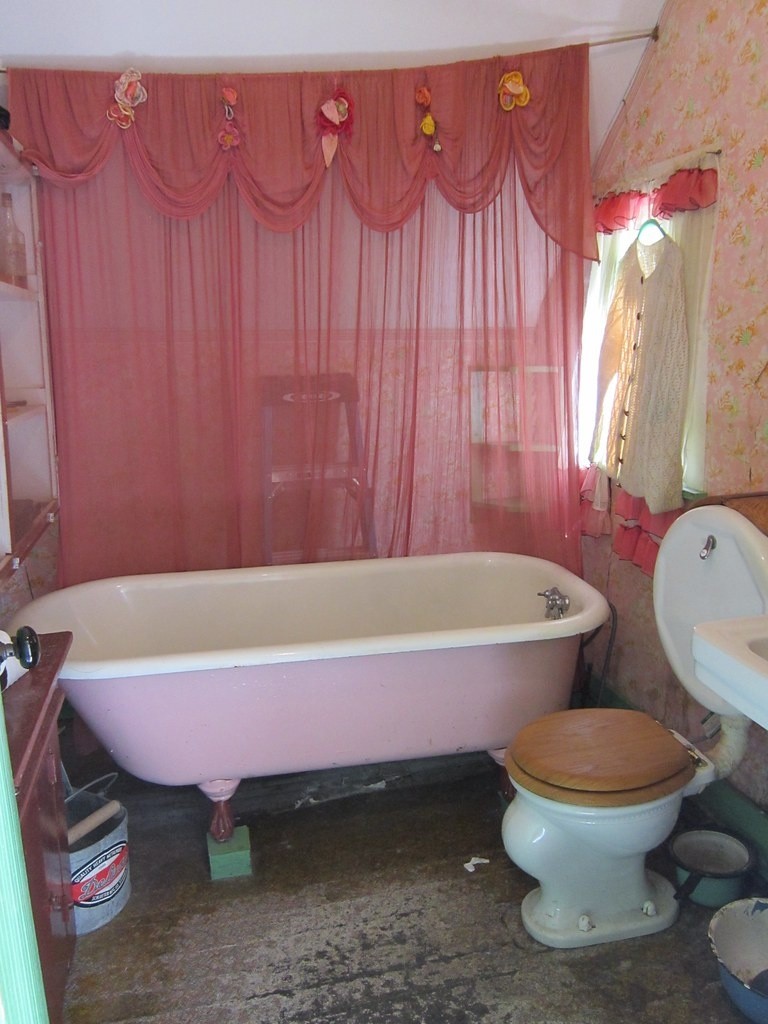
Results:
[690,614,768,732]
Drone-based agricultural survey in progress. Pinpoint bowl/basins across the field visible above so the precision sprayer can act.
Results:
[708,898,768,1024]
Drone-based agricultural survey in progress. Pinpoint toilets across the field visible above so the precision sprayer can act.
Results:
[497,502,768,949]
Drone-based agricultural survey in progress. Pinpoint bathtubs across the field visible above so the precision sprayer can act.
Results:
[13,549,614,802]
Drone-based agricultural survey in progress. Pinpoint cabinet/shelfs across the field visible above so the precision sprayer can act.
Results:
[0,632,90,1024]
[0,129,62,581]
[470,363,564,515]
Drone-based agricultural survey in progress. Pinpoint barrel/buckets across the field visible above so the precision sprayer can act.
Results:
[669,828,754,907]
[43,773,130,938]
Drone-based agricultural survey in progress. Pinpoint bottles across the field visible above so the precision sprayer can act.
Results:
[0,192,27,288]
